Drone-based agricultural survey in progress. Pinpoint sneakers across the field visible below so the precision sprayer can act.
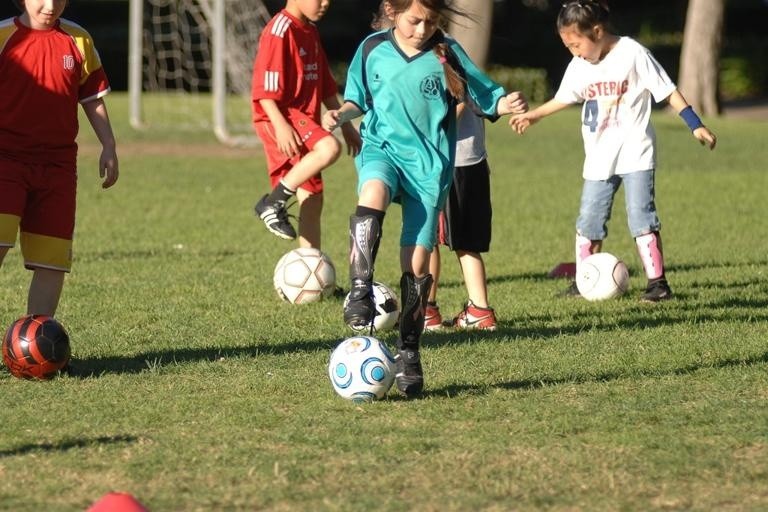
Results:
[343,295,376,333]
[395,351,424,395]
[565,280,581,297]
[423,303,444,329]
[443,301,497,333]
[254,194,296,243]
[641,280,673,301]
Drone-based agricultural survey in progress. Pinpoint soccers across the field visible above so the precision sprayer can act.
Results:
[1,313,70,380]
[327,336,397,402]
[272,247,336,303]
[575,252,628,300]
[343,282,400,332]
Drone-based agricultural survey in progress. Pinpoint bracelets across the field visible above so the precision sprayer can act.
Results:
[678,106,704,134]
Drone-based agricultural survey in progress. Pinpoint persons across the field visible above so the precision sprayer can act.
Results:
[321,0,528,399]
[370,0,497,335]
[249,0,364,302]
[506,0,716,304]
[0,1,118,313]
